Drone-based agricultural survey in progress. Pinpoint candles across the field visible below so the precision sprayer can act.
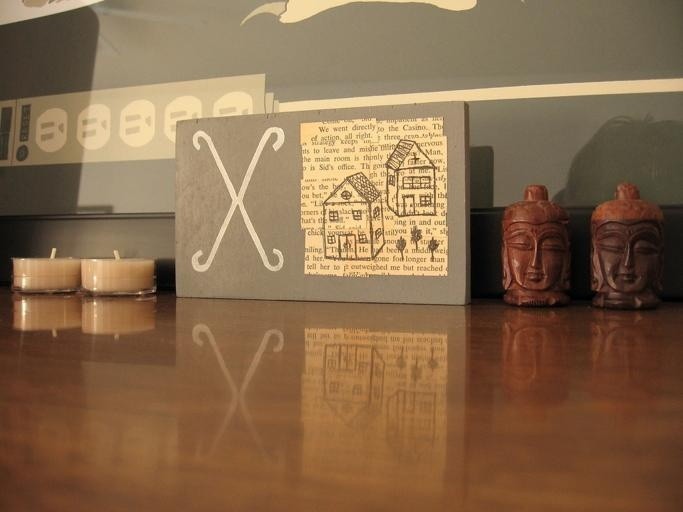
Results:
[12,294,157,344]
[10,247,81,291]
[81,249,155,298]
